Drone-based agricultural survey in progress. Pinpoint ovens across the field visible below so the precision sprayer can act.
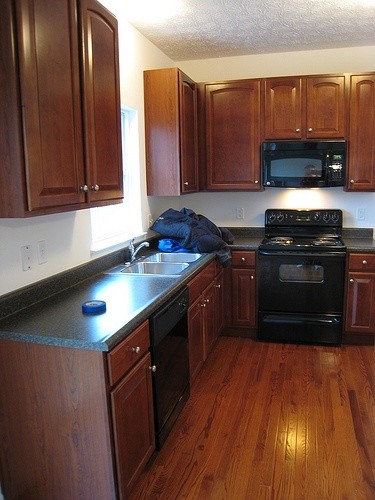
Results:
[257,250,346,347]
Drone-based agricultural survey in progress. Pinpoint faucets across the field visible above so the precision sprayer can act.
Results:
[128,237,150,263]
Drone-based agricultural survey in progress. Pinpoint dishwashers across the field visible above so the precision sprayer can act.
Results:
[149,284,191,451]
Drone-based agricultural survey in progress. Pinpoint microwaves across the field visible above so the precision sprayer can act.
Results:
[259,139,346,187]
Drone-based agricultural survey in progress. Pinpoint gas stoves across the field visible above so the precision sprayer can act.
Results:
[259,208,346,252]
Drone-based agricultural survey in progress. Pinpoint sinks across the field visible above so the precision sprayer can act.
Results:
[104,262,192,278]
[138,249,208,263]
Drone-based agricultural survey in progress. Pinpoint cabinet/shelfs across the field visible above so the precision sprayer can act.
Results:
[0,0,125,220]
[1,319,156,499]
[196,78,264,193]
[177,67,200,198]
[343,252,375,337]
[260,73,348,141]
[223,250,256,332]
[344,71,375,192]
[187,250,224,383]
